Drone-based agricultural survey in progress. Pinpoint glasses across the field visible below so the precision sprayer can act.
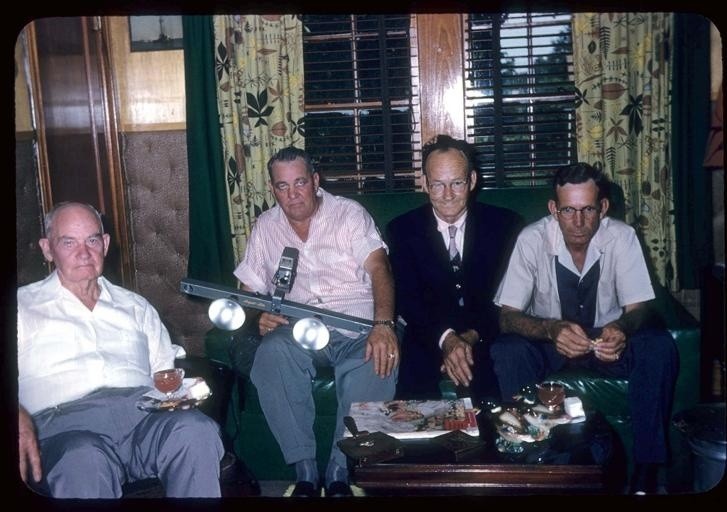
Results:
[554,206,601,220]
[429,181,468,195]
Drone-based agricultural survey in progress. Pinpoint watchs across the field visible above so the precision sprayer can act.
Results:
[374,318,396,328]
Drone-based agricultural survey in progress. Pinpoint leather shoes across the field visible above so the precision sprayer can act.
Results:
[291,481,322,497]
[324,481,354,497]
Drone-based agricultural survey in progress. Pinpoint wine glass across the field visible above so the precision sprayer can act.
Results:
[152,367,183,407]
[535,381,568,421]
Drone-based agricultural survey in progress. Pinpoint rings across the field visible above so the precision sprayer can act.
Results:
[388,350,394,361]
[615,353,619,360]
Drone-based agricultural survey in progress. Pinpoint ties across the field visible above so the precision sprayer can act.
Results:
[449,225,464,306]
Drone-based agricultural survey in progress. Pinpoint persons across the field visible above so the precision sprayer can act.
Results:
[490,161,681,496]
[382,136,522,400]
[18,197,228,499]
[239,146,403,497]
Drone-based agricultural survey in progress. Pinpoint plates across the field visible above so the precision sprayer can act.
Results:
[134,384,215,413]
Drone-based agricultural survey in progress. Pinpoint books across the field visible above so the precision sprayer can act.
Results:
[341,396,479,438]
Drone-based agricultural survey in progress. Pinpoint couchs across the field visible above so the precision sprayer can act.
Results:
[203,185,699,481]
